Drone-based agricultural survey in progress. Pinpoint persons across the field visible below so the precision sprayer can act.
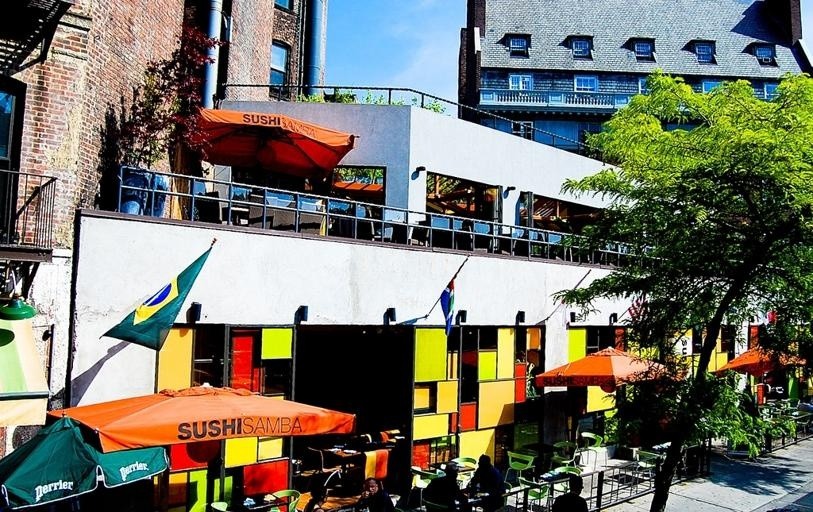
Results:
[354,476,396,511]
[797,394,812,433]
[465,454,505,511]
[301,485,328,512]
[421,461,473,511]
[551,475,588,512]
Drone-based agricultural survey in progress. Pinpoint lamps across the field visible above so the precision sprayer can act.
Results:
[1,269,37,320]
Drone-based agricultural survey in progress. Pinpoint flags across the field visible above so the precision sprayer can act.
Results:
[760,308,778,335]
[628,292,649,321]
[439,280,455,336]
[96,247,212,350]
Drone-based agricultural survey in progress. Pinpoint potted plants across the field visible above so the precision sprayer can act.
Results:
[104,17,222,219]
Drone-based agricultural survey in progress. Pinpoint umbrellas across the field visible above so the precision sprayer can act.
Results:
[48,380,357,501]
[715,343,808,402]
[535,346,685,443]
[0,411,172,511]
[179,105,360,181]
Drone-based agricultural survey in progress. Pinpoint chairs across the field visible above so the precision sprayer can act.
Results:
[449,457,477,483]
[307,429,408,484]
[509,432,604,511]
[411,465,439,511]
[760,403,809,438]
[191,179,407,243]
[781,377,800,407]
[208,488,300,511]
[409,213,638,266]
